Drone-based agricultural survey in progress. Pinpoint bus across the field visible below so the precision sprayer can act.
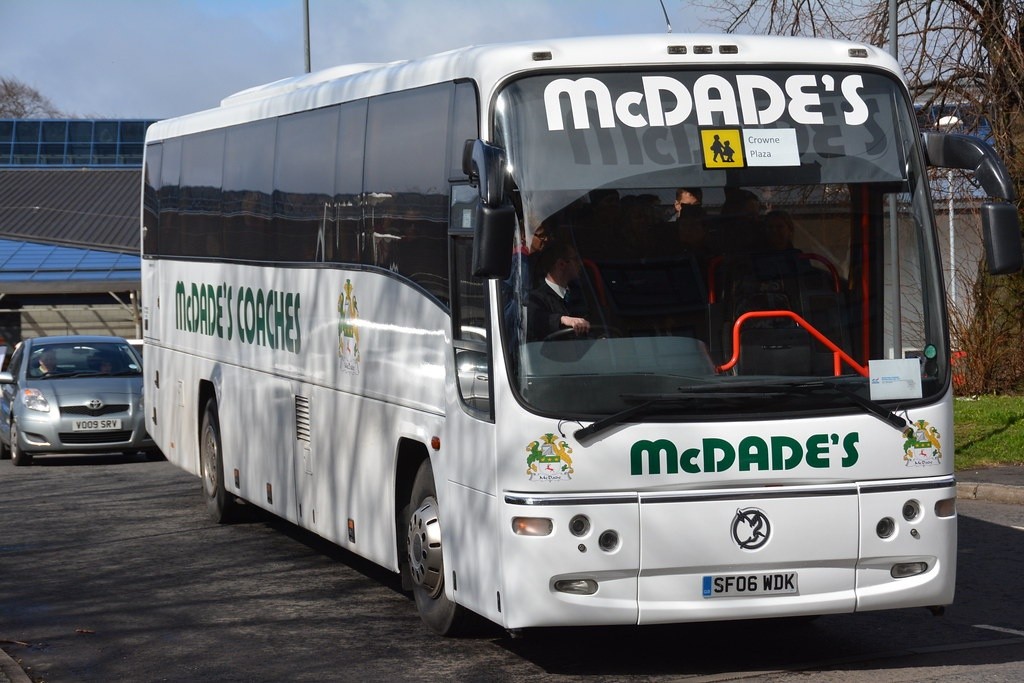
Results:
[141,32,1023,636]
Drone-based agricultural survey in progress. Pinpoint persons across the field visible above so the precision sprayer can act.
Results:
[29,348,65,379]
[370,187,814,374]
[89,353,119,375]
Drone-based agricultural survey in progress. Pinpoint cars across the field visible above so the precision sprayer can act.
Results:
[0,336,165,467]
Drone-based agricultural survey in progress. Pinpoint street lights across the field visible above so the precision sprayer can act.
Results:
[934,114,965,347]
